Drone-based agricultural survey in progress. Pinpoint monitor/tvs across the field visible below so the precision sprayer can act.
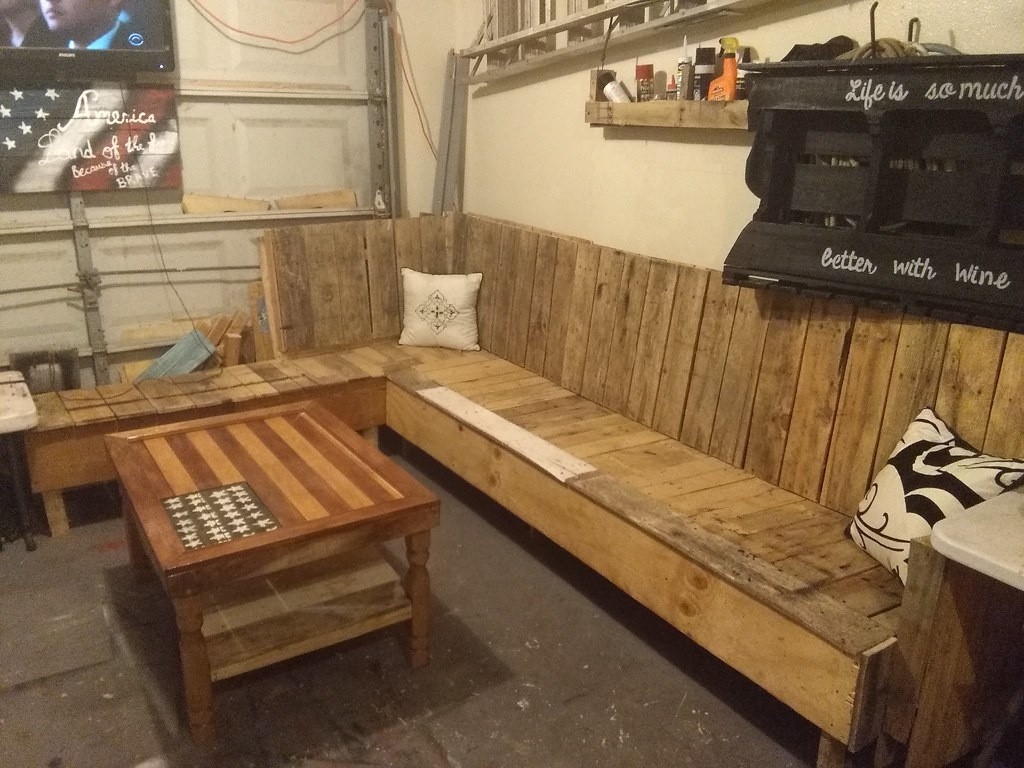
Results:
[0,0,176,73]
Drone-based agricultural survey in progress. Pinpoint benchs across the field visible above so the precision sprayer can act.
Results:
[26,358,388,540]
[262,213,1024,768]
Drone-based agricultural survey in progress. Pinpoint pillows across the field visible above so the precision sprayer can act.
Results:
[844,404,1024,586]
[398,268,482,350]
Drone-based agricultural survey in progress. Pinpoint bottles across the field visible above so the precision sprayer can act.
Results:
[739,47,752,100]
[677,35,695,100]
[665,75,678,100]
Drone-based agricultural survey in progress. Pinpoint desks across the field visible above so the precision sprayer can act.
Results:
[103,399,440,747]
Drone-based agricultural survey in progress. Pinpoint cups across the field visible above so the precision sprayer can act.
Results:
[597,71,631,103]
[693,47,716,100]
[636,63,654,103]
